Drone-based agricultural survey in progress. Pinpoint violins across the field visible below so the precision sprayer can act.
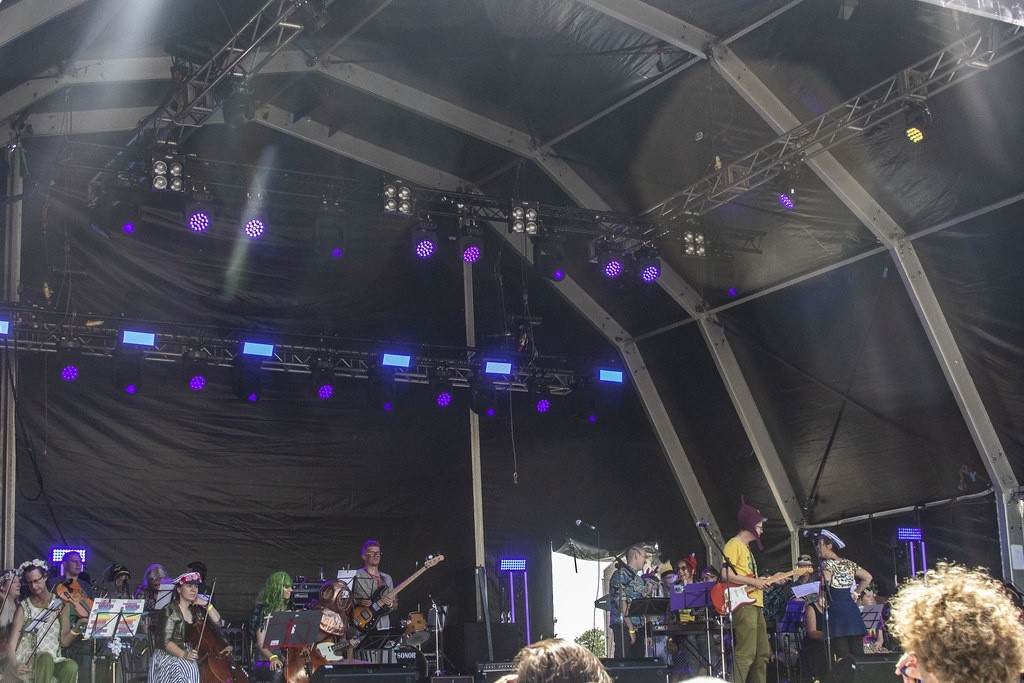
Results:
[56,579,94,619]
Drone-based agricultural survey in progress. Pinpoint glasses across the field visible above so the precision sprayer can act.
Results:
[798,557,811,561]
[366,551,383,557]
[756,525,765,529]
[676,566,690,571]
[26,574,45,584]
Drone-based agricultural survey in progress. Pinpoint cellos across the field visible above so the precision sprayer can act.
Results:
[188,601,251,682]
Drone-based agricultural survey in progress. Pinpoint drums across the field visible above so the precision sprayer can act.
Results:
[367,645,430,683]
[399,611,432,647]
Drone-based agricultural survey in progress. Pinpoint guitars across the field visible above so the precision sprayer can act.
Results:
[348,552,445,633]
[283,634,366,683]
[710,565,814,617]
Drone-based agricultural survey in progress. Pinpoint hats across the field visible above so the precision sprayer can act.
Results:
[171,572,205,587]
[683,552,697,570]
[661,570,679,577]
[815,529,846,550]
[736,504,768,529]
[794,554,814,565]
[104,562,131,583]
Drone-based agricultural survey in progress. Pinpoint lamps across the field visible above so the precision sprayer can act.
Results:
[118,204,139,235]
[574,392,599,425]
[594,241,625,279]
[185,189,213,234]
[112,357,144,395]
[525,384,551,414]
[903,102,934,144]
[310,366,337,400]
[241,193,270,240]
[467,379,496,417]
[779,173,799,210]
[147,150,188,193]
[634,246,661,282]
[182,359,209,391]
[456,219,483,263]
[679,222,710,262]
[380,179,416,215]
[410,219,438,258]
[535,237,565,282]
[367,374,396,411]
[507,196,543,237]
[55,351,80,382]
[424,378,456,411]
[233,367,261,404]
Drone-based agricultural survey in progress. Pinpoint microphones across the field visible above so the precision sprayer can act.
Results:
[803,530,818,537]
[696,522,711,527]
[576,519,598,532]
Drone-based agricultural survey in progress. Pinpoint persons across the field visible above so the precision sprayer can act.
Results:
[885,557,1024,683]
[133,561,234,683]
[250,570,294,683]
[796,601,826,674]
[817,529,873,659]
[352,539,398,630]
[0,551,130,683]
[494,638,614,683]
[722,494,793,683]
[609,544,815,683]
[861,587,884,647]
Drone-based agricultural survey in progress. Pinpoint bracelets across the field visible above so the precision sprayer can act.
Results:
[70,628,79,635]
[269,654,278,660]
[208,604,213,611]
[204,600,209,607]
[668,638,672,641]
[629,629,635,634]
[390,603,393,607]
[852,591,858,597]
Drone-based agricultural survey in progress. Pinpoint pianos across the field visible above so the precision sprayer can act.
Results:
[638,619,776,678]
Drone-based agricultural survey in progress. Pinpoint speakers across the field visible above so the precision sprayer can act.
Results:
[307,663,417,683]
[829,652,905,683]
[472,661,517,683]
[600,656,668,683]
[444,622,519,676]
